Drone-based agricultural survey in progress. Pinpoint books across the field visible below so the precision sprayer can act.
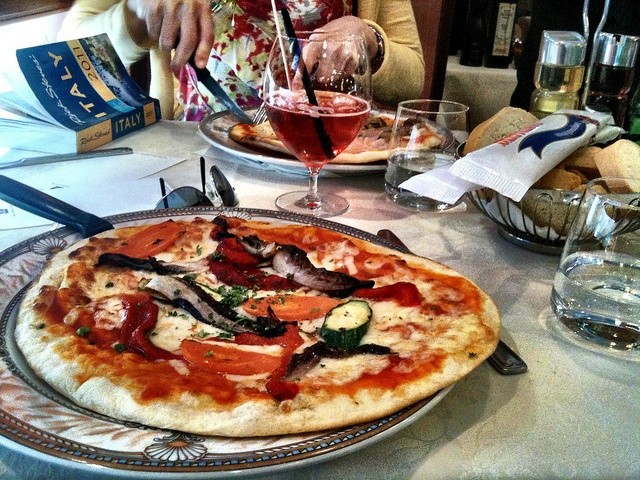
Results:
[0,33,162,156]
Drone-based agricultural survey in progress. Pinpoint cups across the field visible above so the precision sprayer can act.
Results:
[550,178,639,348]
[385,100,470,211]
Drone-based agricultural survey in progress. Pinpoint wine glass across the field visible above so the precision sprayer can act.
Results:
[263,30,373,218]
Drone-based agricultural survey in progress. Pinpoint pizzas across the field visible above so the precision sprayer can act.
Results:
[16,212,504,438]
[227,110,443,164]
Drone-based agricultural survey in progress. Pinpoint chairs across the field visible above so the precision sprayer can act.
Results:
[372,0,441,119]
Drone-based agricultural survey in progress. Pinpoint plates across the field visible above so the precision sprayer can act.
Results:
[199,106,455,174]
[0,206,458,480]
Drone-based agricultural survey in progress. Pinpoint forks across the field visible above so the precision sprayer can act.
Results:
[254,103,268,126]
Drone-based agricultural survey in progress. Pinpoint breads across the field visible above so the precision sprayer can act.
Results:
[552,185,615,238]
[461,105,543,155]
[533,169,583,193]
[513,190,563,225]
[595,134,639,194]
[569,146,601,169]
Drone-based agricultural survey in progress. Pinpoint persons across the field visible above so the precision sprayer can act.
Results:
[509,1,640,132]
[53,0,426,120]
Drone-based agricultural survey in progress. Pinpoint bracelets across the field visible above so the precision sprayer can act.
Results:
[373,28,384,68]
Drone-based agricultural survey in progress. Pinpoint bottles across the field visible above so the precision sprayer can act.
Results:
[590,33,640,141]
[484,1,519,69]
[459,0,490,67]
[527,30,587,116]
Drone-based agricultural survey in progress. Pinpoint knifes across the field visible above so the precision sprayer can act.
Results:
[0,147,133,170]
[173,40,254,124]
[377,229,527,374]
